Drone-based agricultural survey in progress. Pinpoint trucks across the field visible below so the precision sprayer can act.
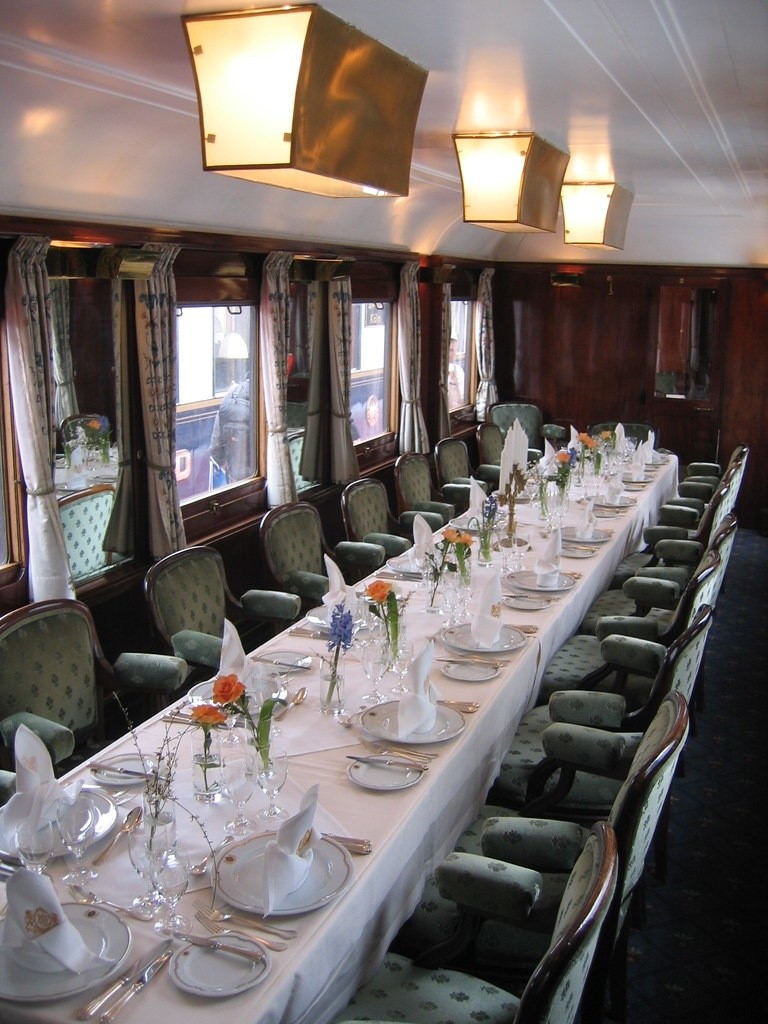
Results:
[173,301,482,498]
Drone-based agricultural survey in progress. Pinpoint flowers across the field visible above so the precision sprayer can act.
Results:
[212,657,282,726]
[323,603,354,656]
[62,414,110,444]
[429,527,457,571]
[365,580,410,625]
[553,445,578,481]
[104,687,222,906]
[524,450,556,483]
[189,707,225,744]
[455,533,475,574]
[595,429,616,452]
[572,432,594,456]
[469,494,504,529]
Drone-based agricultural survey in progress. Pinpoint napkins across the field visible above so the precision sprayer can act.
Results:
[535,526,564,588]
[566,423,582,454]
[1,726,86,861]
[465,476,490,523]
[211,616,282,716]
[604,468,624,504]
[630,442,645,481]
[396,639,439,739]
[644,430,657,463]
[613,423,634,450]
[409,514,440,572]
[0,867,118,980]
[318,553,363,622]
[576,495,598,540]
[540,437,556,473]
[252,782,323,920]
[464,565,505,648]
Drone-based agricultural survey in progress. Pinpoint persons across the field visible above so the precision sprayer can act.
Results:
[448,338,464,410]
[210,379,250,484]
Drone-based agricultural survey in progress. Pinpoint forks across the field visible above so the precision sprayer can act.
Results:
[194,910,289,952]
[191,897,298,951]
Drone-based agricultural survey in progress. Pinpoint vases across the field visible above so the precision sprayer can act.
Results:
[99,433,111,463]
[81,443,96,471]
[535,482,551,521]
[426,569,445,616]
[379,615,407,672]
[193,733,222,802]
[598,445,617,477]
[474,526,494,568]
[573,456,589,486]
[319,655,345,713]
[552,477,571,515]
[141,788,174,865]
[453,564,474,603]
[63,443,73,469]
[243,715,273,779]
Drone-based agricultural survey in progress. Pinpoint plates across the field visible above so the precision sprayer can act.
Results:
[385,555,433,578]
[449,517,482,531]
[560,526,612,542]
[305,606,373,629]
[209,829,354,915]
[0,788,119,862]
[580,494,638,507]
[168,932,272,997]
[560,546,598,558]
[502,597,552,610]
[441,661,500,682]
[346,754,424,791]
[359,699,467,744]
[187,680,289,716]
[441,623,527,652]
[256,651,312,673]
[0,901,132,1002]
[505,569,576,592]
[90,752,157,786]
[609,472,655,483]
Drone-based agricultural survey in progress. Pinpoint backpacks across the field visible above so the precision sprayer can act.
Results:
[217,382,251,481]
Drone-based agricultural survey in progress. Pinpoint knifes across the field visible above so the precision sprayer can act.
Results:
[87,761,166,780]
[346,756,429,771]
[74,938,180,1024]
[171,932,266,964]
[374,571,421,582]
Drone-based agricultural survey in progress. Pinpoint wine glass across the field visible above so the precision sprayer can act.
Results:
[359,638,415,704]
[497,532,531,576]
[254,747,289,822]
[127,820,169,913]
[538,491,571,535]
[148,845,192,937]
[15,796,103,891]
[439,572,476,628]
[222,752,259,836]
[593,434,672,485]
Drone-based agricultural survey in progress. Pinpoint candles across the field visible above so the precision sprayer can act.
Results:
[503,437,510,489]
[497,448,506,494]
[505,427,514,475]
[513,416,521,469]
[518,429,526,479]
[522,437,529,484]
[516,427,523,475]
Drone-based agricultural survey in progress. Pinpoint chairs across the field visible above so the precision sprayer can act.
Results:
[59,485,121,580]
[0,401,749,1024]
[56,414,101,456]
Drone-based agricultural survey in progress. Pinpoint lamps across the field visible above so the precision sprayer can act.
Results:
[558,183,636,252]
[184,5,427,198]
[450,133,566,233]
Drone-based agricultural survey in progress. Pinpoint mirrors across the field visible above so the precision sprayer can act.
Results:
[655,279,729,403]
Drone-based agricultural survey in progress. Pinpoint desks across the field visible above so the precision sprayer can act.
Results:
[50,446,121,500]
[0,443,680,1023]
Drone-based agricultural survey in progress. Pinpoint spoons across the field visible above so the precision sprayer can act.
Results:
[274,687,308,721]
[66,883,153,921]
[187,835,234,876]
